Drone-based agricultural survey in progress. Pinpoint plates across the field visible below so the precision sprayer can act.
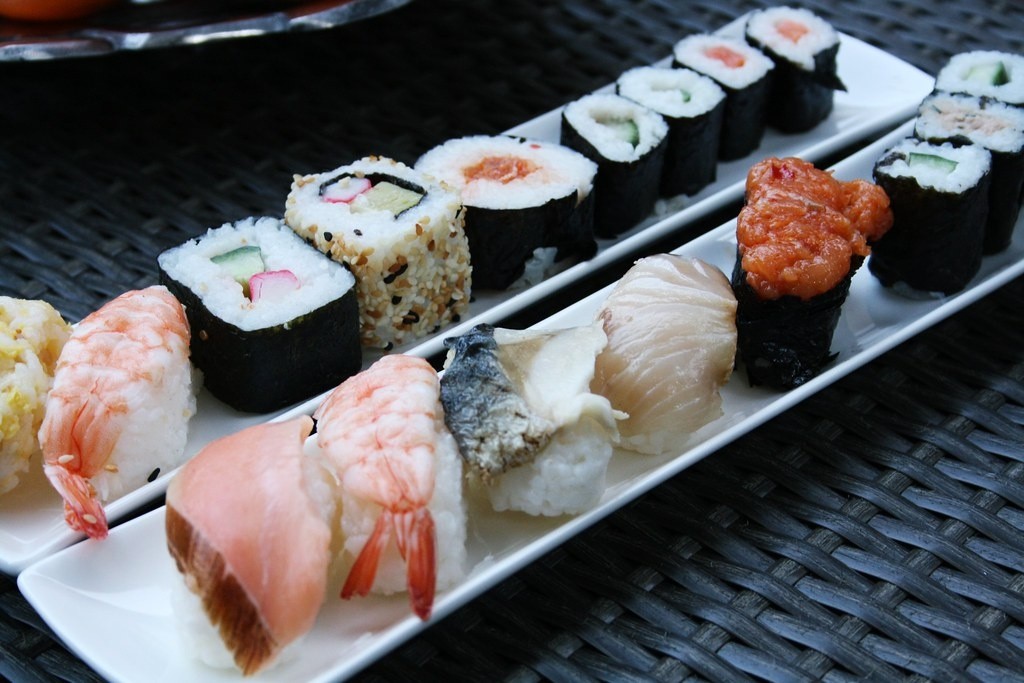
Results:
[17,114,1024,683]
[0,16,935,575]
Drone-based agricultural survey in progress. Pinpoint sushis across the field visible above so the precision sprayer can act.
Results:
[0,5,1024,674]
